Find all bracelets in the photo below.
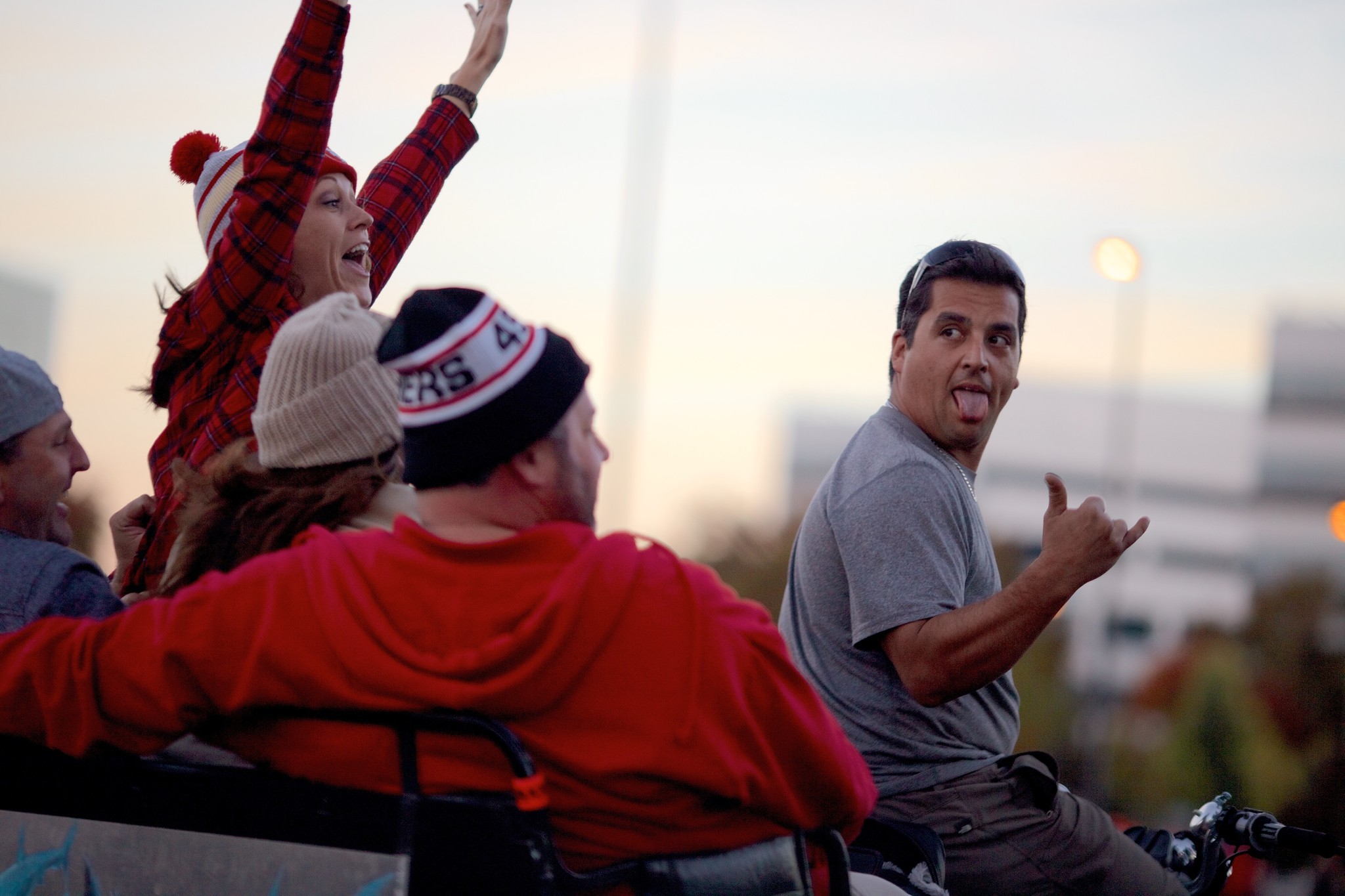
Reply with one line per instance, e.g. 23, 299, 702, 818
431, 83, 478, 118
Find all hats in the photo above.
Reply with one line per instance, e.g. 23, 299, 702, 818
252, 292, 409, 466
0, 349, 62, 442
378, 285, 589, 484
168, 131, 357, 259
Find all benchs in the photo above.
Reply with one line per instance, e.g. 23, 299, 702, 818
0, 701, 853, 896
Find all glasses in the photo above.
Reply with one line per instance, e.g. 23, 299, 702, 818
902, 242, 1025, 329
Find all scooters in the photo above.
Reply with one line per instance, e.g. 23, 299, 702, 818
0, 701, 1345, 896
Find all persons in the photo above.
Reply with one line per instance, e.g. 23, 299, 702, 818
775, 241, 1195, 896
121, 0, 514, 595
1, 348, 125, 632
0, 288, 879, 896
156, 293, 407, 596
